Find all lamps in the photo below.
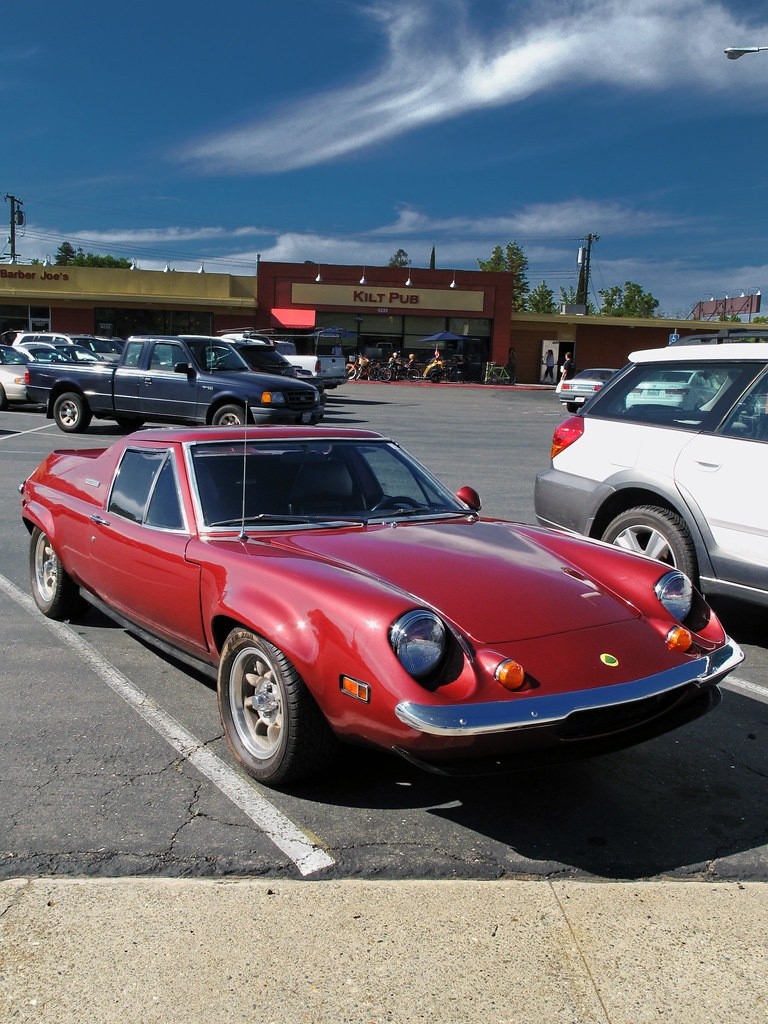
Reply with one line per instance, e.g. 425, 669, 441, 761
405, 268, 413, 287
129, 257, 139, 272
449, 270, 458, 289
197, 261, 206, 275
315, 264, 324, 282
163, 260, 171, 274
358, 266, 368, 285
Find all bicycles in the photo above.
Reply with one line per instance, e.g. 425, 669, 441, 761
480, 363, 516, 386
346, 353, 470, 386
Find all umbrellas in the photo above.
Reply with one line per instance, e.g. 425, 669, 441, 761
311, 324, 357, 355
419, 330, 480, 361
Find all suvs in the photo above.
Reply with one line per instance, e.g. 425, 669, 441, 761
535, 343, 768, 635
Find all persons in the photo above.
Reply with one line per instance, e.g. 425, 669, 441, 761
359, 357, 370, 381
539, 350, 555, 385
560, 352, 574, 403
388, 352, 402, 380
508, 346, 517, 377
3, 326, 17, 347
332, 342, 342, 355
406, 353, 415, 383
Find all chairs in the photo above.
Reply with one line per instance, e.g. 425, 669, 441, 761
284, 460, 368, 516
154, 462, 220, 523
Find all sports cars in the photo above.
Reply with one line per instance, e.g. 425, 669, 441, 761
17, 421, 747, 786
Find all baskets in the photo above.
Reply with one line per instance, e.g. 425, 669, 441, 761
349, 356, 358, 362
359, 357, 369, 366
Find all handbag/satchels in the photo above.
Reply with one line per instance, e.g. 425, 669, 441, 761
555, 377, 564, 394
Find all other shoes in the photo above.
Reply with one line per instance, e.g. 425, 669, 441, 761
562, 402, 567, 406
539, 380, 545, 383
547, 381, 553, 385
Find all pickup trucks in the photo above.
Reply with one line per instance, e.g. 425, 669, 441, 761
25, 335, 325, 434
213, 336, 350, 391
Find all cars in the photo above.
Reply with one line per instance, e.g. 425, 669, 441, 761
0, 328, 160, 372
0, 345, 33, 410
558, 368, 623, 413
625, 368, 722, 417
